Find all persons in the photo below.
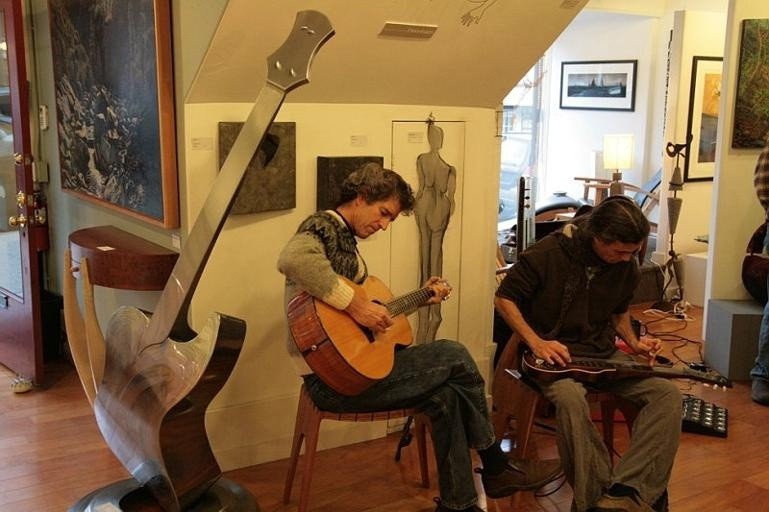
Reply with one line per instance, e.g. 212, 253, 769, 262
412, 124, 457, 345
748, 300, 769, 404
494, 197, 683, 511
276, 160, 563, 512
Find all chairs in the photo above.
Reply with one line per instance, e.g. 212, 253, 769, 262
502, 163, 661, 263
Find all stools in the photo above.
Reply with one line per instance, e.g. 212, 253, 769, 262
282, 385, 434, 512
492, 367, 644, 512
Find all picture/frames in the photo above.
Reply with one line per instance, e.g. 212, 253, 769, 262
559, 60, 637, 112
47, 0, 182, 232
683, 56, 724, 182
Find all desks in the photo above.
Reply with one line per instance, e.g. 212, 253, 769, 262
63, 224, 177, 407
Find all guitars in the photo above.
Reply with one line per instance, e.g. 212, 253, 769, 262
288, 276, 451, 396
94, 10, 334, 511
522, 346, 734, 390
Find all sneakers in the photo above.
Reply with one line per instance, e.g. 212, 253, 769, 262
432, 496, 484, 512
751, 379, 769, 404
474, 457, 561, 499
